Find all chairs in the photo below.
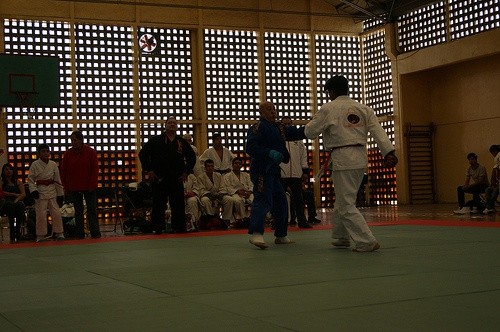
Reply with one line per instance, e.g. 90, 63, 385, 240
95, 186, 125, 234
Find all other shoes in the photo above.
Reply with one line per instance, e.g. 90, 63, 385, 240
308, 218, 322, 224
124, 217, 274, 234
298, 221, 312, 228
291, 221, 297, 226
91, 232, 101, 238
37, 236, 47, 241
53, 233, 65, 240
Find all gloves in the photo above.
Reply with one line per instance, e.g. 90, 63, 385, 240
32, 191, 39, 199
57, 196, 63, 207
385, 153, 398, 168
269, 149, 284, 164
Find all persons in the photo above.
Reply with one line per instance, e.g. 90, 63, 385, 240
59, 131, 101, 239
182, 173, 198, 232
196, 159, 234, 228
0, 164, 27, 243
482, 145, 500, 213
181, 134, 198, 165
304, 75, 398, 252
246, 100, 307, 250
28, 144, 65, 241
138, 117, 196, 234
198, 133, 235, 177
223, 157, 272, 228
287, 169, 321, 225
278, 117, 313, 227
453, 153, 489, 213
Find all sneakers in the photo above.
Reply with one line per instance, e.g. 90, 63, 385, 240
353, 241, 380, 252
481, 208, 496, 214
275, 236, 296, 244
470, 207, 480, 213
453, 207, 465, 214
250, 233, 268, 250
330, 239, 351, 248
